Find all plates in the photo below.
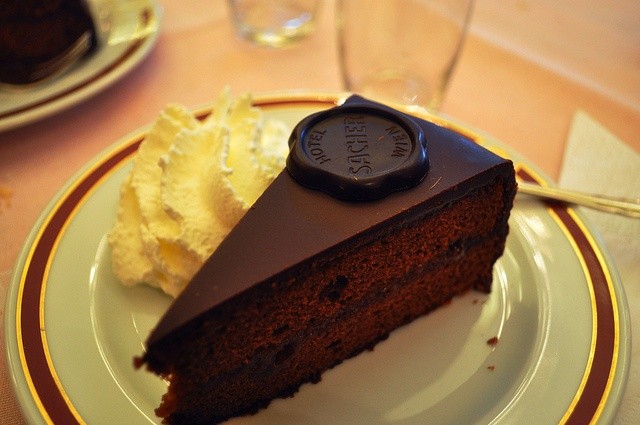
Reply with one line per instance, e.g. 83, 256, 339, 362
0, 0, 163, 135
6, 90, 633, 425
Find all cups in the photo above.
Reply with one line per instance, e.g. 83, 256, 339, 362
334, 1, 473, 118
231, 1, 326, 50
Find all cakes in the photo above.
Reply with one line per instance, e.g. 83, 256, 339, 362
148, 92, 517, 425
